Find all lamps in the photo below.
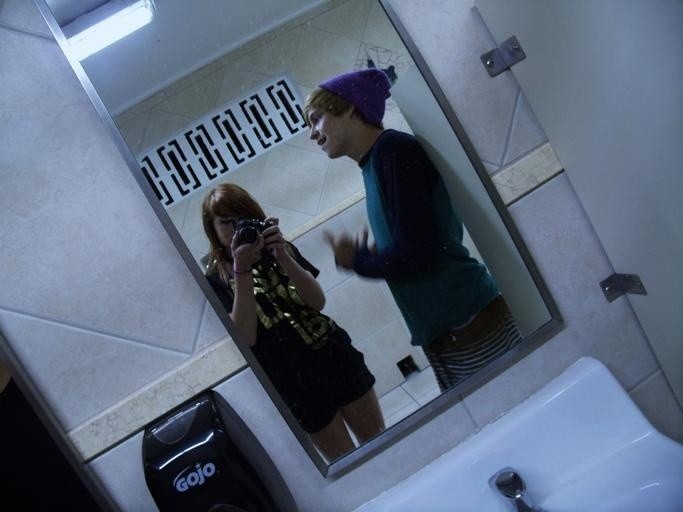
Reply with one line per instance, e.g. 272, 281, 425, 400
60, 0, 155, 63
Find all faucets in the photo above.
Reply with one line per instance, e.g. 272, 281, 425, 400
487, 466, 540, 510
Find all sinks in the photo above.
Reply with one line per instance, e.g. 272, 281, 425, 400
348, 356, 682, 511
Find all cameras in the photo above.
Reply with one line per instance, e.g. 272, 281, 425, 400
235, 221, 270, 247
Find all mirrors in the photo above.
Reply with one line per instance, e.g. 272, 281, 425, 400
33, 0, 565, 482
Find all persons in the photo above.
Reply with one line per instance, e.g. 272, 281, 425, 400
202, 183, 386, 463
301, 65, 524, 394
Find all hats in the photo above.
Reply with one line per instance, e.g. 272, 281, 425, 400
319, 68, 391, 123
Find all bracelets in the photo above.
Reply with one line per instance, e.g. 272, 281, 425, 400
233, 267, 253, 274
